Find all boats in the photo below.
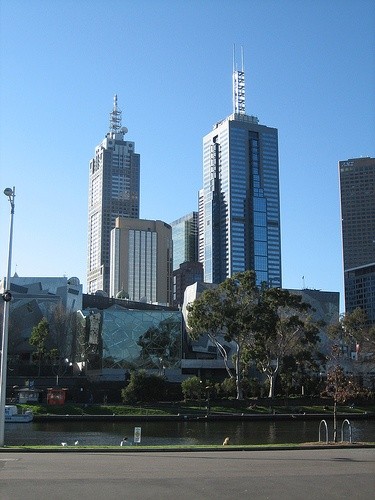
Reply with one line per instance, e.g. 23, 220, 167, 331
5, 405, 35, 422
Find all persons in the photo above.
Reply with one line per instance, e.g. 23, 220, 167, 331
120, 437, 129, 446
222, 435, 232, 445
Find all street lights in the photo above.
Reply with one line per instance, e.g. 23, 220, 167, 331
1, 185, 16, 448
